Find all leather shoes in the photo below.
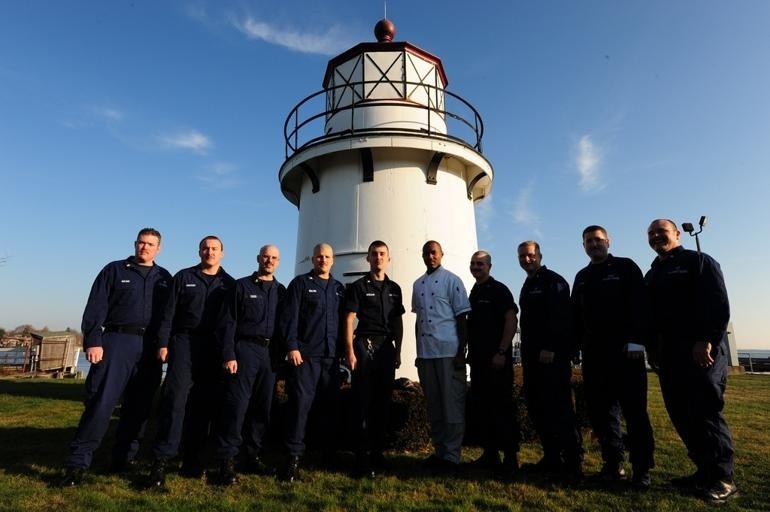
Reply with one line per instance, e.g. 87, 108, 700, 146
60, 456, 304, 490
590, 463, 740, 505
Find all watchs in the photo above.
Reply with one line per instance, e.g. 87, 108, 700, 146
498, 348, 506, 355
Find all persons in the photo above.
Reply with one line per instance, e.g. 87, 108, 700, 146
517, 242, 582, 483
62, 227, 174, 489
218, 243, 287, 485
643, 218, 738, 506
570, 226, 655, 488
344, 242, 405, 479
411, 241, 474, 475
463, 250, 518, 480
137, 235, 236, 491
280, 242, 347, 483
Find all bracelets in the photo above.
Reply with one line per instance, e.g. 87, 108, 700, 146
500, 343, 508, 352
